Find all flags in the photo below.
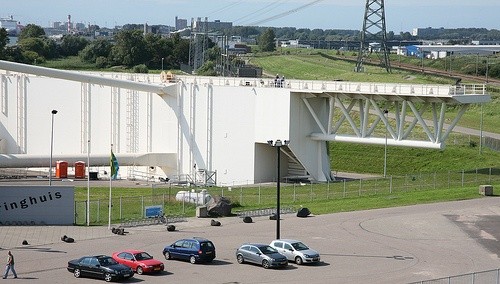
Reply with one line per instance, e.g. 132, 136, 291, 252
110, 147, 120, 180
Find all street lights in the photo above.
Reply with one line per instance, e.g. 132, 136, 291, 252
266, 140, 290, 238
383, 109, 389, 178
48, 110, 58, 185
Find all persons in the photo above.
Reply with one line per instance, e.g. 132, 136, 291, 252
3, 251, 17, 279
274, 74, 285, 87
456, 78, 462, 88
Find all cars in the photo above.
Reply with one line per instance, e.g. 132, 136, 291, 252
162, 237, 217, 265
66, 255, 135, 282
269, 239, 321, 265
236, 243, 288, 270
111, 249, 165, 274
